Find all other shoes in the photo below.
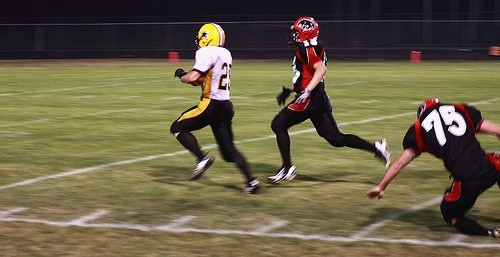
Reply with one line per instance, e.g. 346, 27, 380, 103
488, 227, 500, 238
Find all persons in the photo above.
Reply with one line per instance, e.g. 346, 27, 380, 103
366, 98, 500, 238
267, 16, 392, 184
170, 24, 262, 194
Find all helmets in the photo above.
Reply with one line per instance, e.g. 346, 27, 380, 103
197, 23, 225, 48
417, 98, 439, 119
289, 16, 319, 43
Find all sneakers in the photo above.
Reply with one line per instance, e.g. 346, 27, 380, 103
374, 138, 391, 167
241, 176, 263, 194
267, 164, 297, 186
188, 151, 214, 181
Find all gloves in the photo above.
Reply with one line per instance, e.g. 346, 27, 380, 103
276, 86, 291, 107
295, 88, 311, 105
174, 68, 187, 78
